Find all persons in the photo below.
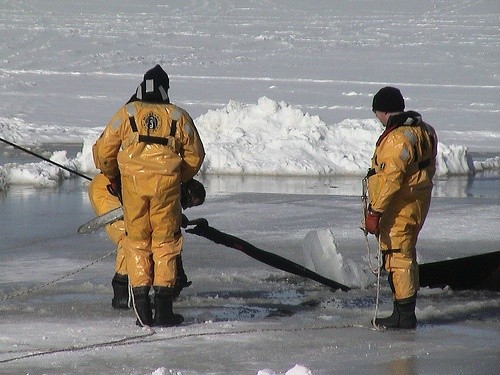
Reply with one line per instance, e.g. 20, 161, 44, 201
92, 64, 205, 327
88, 172, 206, 309
365, 86, 438, 330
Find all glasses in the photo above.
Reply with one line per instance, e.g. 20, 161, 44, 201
372, 109, 377, 114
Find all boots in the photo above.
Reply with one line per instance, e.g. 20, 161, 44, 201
132, 286, 154, 327
371, 292, 417, 329
154, 286, 184, 327
112, 273, 129, 309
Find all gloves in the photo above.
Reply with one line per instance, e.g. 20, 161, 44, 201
365, 209, 382, 237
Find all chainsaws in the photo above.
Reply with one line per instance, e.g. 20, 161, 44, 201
78, 178, 206, 236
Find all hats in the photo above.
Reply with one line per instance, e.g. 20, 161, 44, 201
373, 87, 405, 113
144, 64, 170, 89
